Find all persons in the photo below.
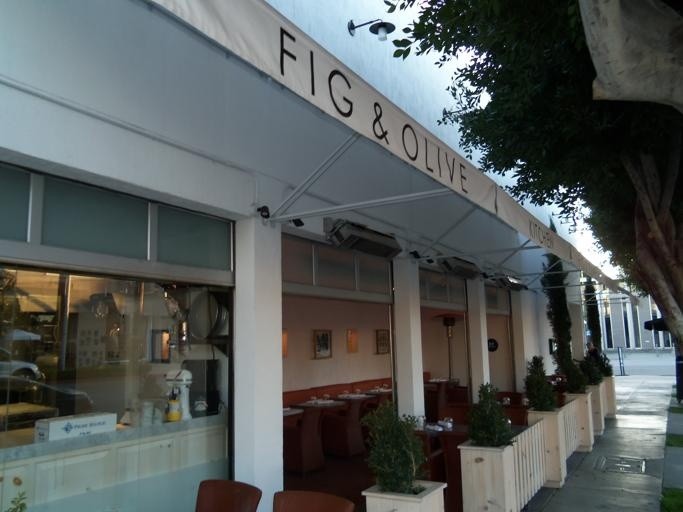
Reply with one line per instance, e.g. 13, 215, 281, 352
585, 343, 599, 359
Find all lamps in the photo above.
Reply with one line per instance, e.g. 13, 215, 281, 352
323, 217, 528, 292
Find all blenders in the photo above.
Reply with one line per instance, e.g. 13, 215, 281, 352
164, 369, 192, 420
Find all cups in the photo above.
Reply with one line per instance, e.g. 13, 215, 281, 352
555, 377, 561, 382
443, 416, 453, 431
502, 397, 512, 405
411, 415, 426, 431
521, 397, 529, 407
165, 400, 181, 422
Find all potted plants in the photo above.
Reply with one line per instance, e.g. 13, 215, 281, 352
359, 400, 447, 512
457, 349, 616, 512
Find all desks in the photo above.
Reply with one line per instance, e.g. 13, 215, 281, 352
1, 402, 59, 451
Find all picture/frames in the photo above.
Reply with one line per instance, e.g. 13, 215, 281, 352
375, 329, 390, 354
313, 329, 332, 359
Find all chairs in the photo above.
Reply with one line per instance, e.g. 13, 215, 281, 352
437, 431, 470, 512
196, 480, 355, 512
